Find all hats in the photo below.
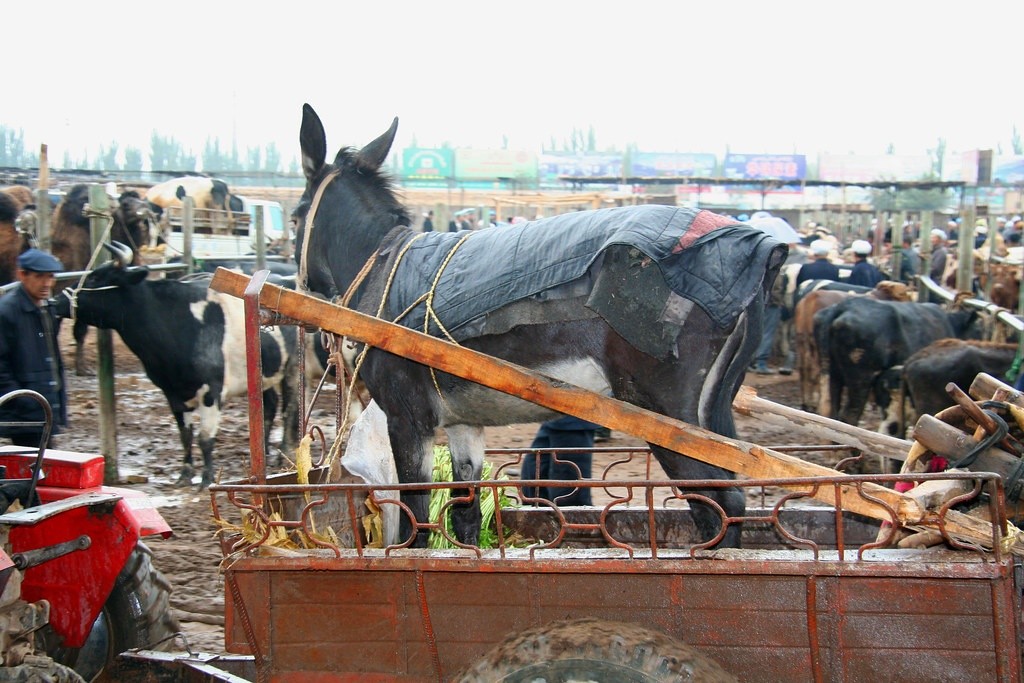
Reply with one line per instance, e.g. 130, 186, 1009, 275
19, 249, 66, 272
931, 229, 948, 242
810, 239, 832, 255
996, 217, 1007, 223
464, 208, 475, 214
975, 226, 987, 234
488, 210, 496, 216
453, 210, 463, 217
975, 218, 988, 227
851, 239, 873, 257
1012, 216, 1021, 223
428, 210, 434, 216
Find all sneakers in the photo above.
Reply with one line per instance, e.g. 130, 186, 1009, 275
755, 367, 774, 374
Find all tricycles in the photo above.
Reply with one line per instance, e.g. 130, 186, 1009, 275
0, 269, 1024, 683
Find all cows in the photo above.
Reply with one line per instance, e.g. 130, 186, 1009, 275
770, 252, 1024, 466
46, 239, 360, 490
143, 175, 243, 250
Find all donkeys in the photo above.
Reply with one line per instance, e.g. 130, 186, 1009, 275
0, 182, 162, 295
289, 101, 779, 549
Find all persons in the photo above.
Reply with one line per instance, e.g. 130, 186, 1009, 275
422, 209, 435, 232
518, 411, 603, 506
795, 238, 840, 290
446, 209, 516, 232
868, 214, 1024, 291
848, 239, 888, 287
0, 249, 68, 449
